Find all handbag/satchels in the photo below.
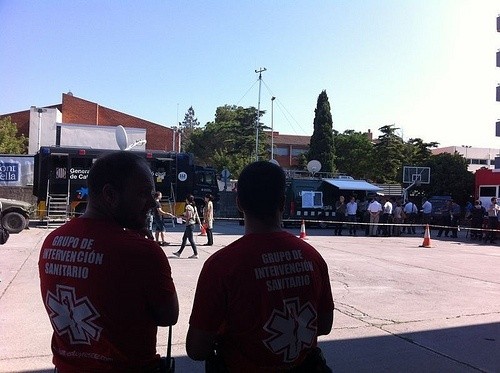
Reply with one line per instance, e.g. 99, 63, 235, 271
401, 211, 407, 218
411, 212, 418, 218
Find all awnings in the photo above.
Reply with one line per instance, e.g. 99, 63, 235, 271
322, 178, 384, 191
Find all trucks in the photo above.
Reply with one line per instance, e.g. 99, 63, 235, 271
285, 177, 385, 227
33, 144, 219, 224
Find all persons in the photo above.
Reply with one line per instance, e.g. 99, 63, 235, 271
334, 194, 500, 244
198, 194, 215, 246
186, 161, 335, 373
153, 191, 173, 245
40, 151, 178, 373
173, 194, 204, 258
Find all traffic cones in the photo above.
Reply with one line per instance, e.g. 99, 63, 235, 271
419, 224, 432, 248
197, 223, 207, 236
299, 220, 309, 240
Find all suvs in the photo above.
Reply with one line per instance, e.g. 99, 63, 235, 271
0, 194, 36, 234
424, 195, 454, 224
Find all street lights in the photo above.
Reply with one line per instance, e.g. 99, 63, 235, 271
270, 96, 277, 159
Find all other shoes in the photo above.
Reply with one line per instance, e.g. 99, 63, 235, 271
338, 233, 342, 236
413, 232, 415, 234
162, 242, 170, 246
490, 240, 496, 244
334, 233, 337, 236
445, 235, 449, 237
173, 252, 180, 256
484, 240, 487, 243
408, 232, 411, 234
450, 235, 457, 238
437, 235, 441, 237
188, 255, 199, 259
204, 244, 212, 246
